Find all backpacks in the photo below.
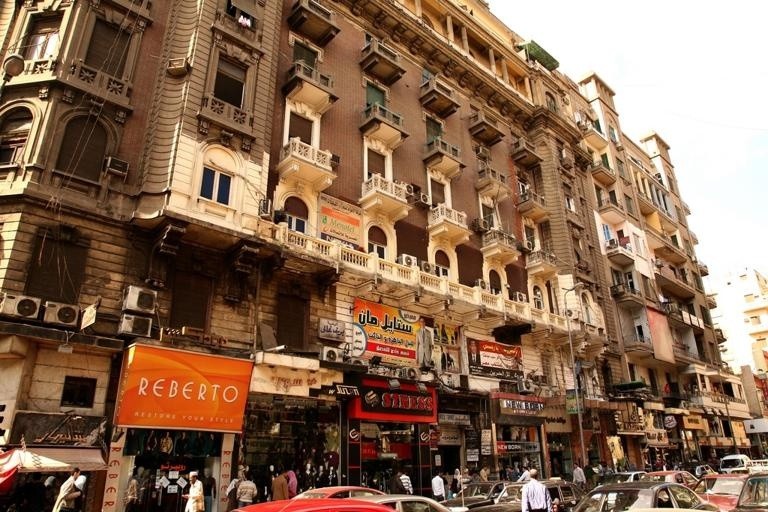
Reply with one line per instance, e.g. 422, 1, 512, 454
389, 474, 407, 494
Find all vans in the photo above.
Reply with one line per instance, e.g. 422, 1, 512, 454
720, 454, 751, 474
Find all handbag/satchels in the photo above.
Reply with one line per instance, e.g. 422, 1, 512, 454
229, 487, 236, 500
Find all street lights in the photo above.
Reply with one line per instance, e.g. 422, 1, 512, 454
715, 349, 740, 454
564, 282, 586, 469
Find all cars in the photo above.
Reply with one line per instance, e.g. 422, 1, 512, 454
229, 485, 451, 512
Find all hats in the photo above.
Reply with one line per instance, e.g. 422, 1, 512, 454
189, 470, 199, 477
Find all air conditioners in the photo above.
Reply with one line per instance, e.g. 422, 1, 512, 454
520, 238, 535, 253
473, 277, 491, 293
476, 145, 490, 158
415, 190, 434, 208
106, 155, 130, 178
420, 258, 436, 275
0, 284, 158, 338
397, 253, 420, 269
607, 239, 677, 313
322, 345, 534, 393
167, 57, 189, 75
474, 216, 491, 233
397, 181, 414, 198
511, 290, 528, 304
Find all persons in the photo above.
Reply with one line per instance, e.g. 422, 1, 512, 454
224, 463, 338, 507
431, 466, 539, 501
572, 462, 586, 489
201, 468, 216, 512
655, 457, 686, 471
182, 471, 206, 512
390, 469, 414, 496
37, 468, 87, 511
585, 460, 631, 482
522, 470, 553, 512
